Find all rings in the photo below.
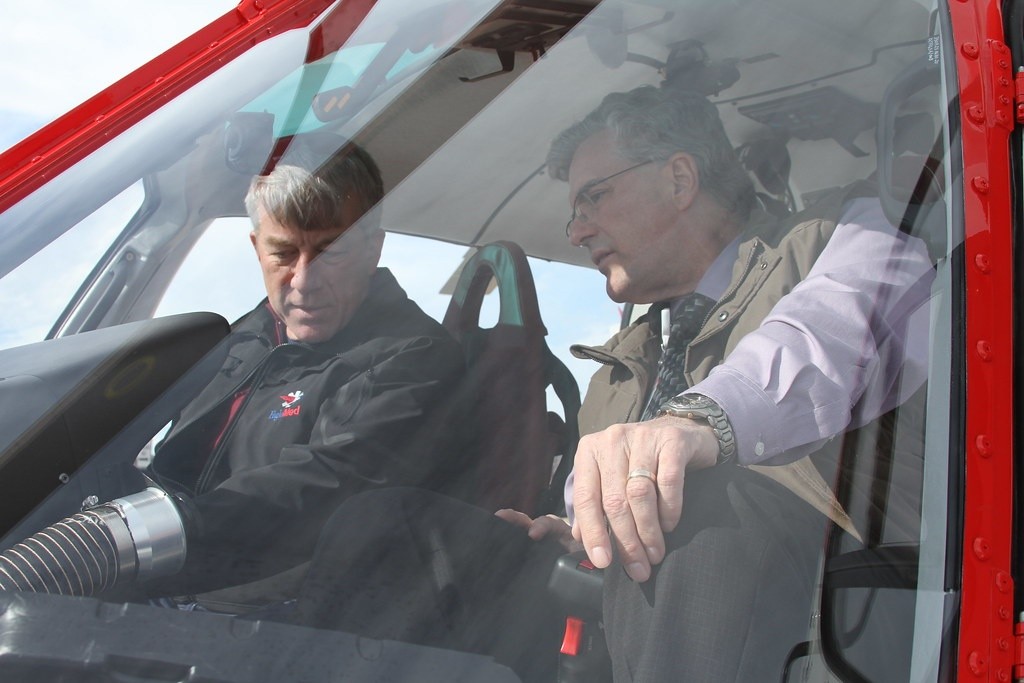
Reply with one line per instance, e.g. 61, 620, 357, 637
626, 470, 657, 483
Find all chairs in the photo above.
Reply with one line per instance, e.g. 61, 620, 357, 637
421, 240, 581, 520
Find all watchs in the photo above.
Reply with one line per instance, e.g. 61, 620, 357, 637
656, 393, 737, 464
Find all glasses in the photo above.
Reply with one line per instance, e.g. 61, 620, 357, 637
566, 160, 657, 237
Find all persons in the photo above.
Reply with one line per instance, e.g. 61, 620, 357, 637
289, 85, 937, 683
60, 130, 484, 626
